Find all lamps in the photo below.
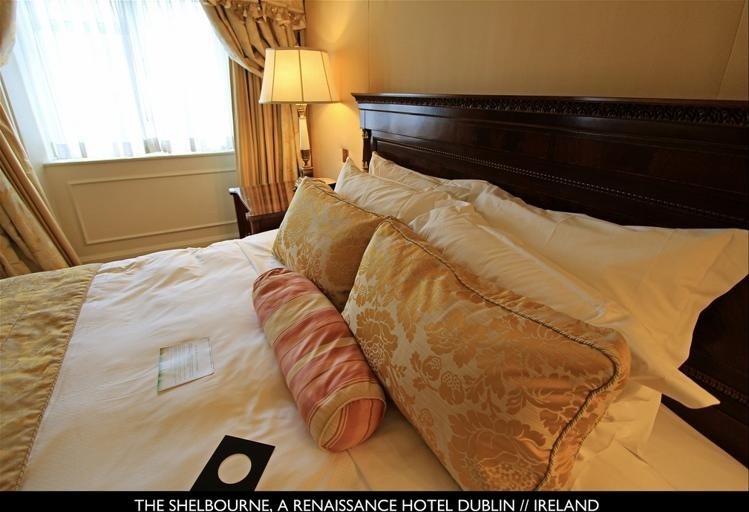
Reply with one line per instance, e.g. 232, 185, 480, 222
256, 47, 339, 186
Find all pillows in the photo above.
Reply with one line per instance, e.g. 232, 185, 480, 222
368, 150, 487, 203
465, 177, 748, 466
250, 264, 386, 453
271, 176, 384, 314
342, 222, 632, 491
330, 157, 454, 225
402, 194, 723, 491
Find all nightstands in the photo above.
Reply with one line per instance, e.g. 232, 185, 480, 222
226, 179, 296, 240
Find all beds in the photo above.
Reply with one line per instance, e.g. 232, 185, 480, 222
1, 94, 747, 490
